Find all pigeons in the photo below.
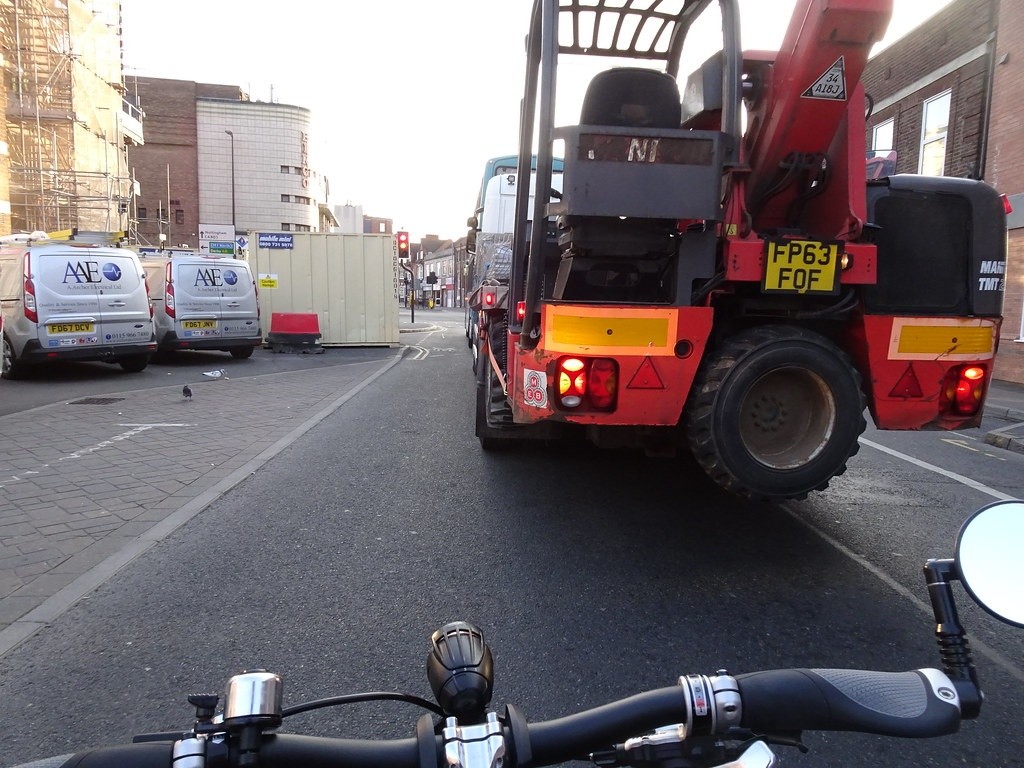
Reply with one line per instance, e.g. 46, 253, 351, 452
182, 384, 192, 401
201, 368, 226, 380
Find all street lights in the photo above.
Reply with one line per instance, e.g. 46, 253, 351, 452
225, 129, 235, 225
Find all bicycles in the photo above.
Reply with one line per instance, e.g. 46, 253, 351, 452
11, 504, 1024, 768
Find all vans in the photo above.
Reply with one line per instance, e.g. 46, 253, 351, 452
135, 251, 263, 358
0, 242, 158, 380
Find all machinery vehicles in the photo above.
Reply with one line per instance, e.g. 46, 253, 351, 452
465, 0, 1005, 503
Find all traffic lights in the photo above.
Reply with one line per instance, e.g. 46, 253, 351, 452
436, 277, 438, 283
427, 276, 430, 284
404, 277, 406, 284
398, 232, 408, 257
